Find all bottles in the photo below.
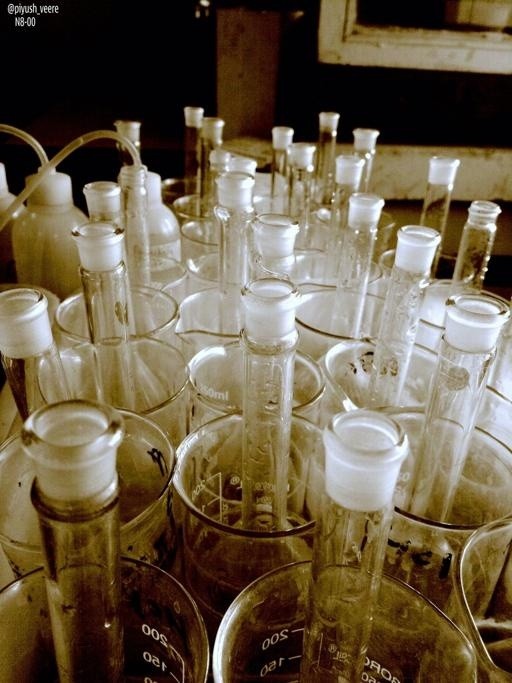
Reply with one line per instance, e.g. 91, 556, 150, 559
0, 99, 512, 681
